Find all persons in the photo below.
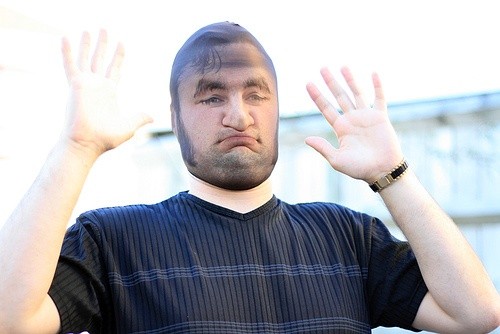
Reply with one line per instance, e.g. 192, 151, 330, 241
1, 19, 499, 333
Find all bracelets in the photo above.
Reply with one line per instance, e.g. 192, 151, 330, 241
370, 155, 409, 195
